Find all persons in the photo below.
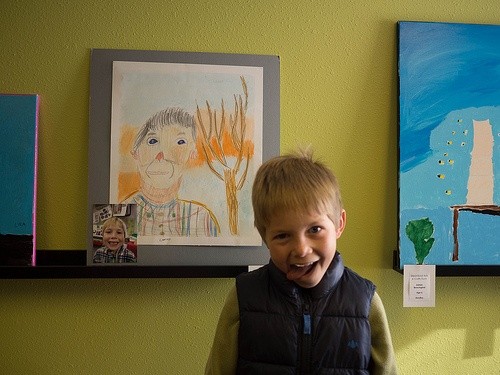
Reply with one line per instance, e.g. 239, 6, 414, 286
92, 216, 136, 263
204, 138, 394, 375
